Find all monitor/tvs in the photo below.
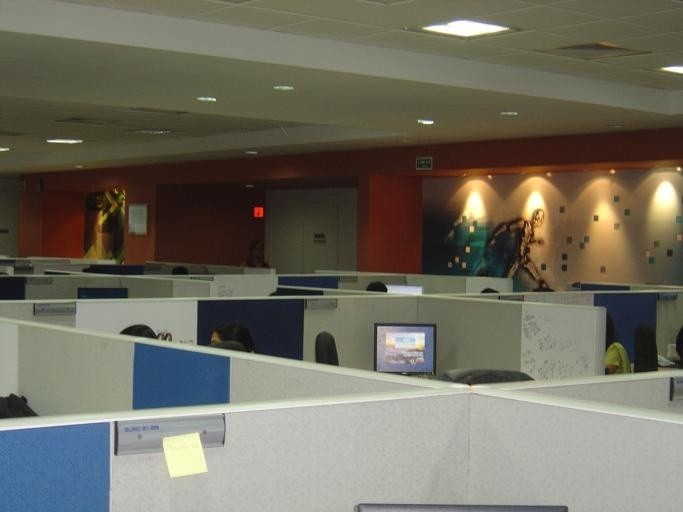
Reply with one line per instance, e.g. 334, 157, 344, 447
77, 287, 127, 299
0, 277, 27, 300
373, 321, 436, 375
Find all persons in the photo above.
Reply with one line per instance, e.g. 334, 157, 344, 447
210, 322, 256, 353
172, 266, 190, 275
481, 287, 499, 293
241, 238, 271, 269
366, 281, 387, 292
120, 324, 157, 339
487, 209, 550, 289
604, 312, 632, 375
